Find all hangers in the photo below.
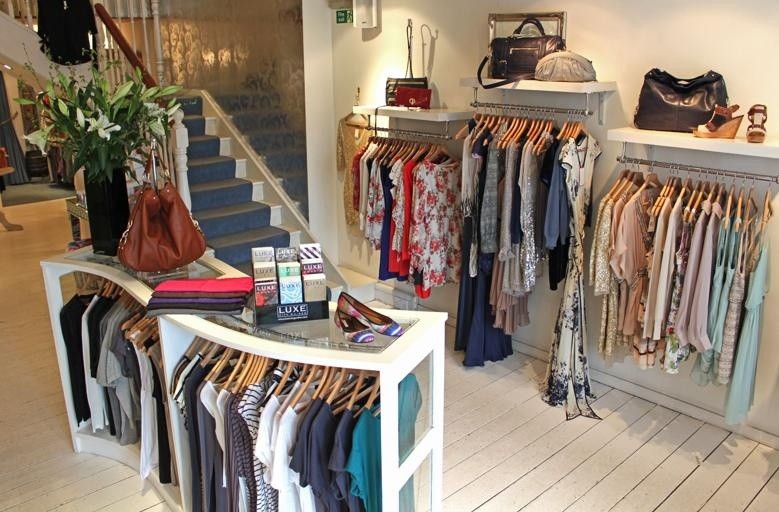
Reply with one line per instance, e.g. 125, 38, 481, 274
76, 274, 160, 359
343, 87, 368, 130
607, 156, 775, 236
365, 130, 451, 165
454, 102, 589, 157
166, 335, 379, 416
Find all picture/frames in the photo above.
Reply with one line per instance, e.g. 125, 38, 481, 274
488, 11, 567, 77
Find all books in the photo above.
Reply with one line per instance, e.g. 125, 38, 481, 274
251, 243, 327, 307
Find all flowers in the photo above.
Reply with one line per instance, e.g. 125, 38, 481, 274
6, 30, 184, 183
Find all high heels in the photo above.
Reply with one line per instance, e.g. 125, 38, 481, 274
692, 102, 744, 139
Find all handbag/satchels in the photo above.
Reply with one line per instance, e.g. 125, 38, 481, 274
116, 147, 209, 275
632, 62, 731, 135
382, 74, 433, 112
474, 16, 598, 89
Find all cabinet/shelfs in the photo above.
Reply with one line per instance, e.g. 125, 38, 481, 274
38, 240, 449, 511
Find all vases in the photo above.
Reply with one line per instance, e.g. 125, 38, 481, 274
83, 169, 136, 254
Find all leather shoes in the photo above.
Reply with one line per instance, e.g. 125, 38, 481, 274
332, 291, 404, 346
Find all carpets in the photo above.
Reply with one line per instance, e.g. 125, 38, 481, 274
2, 181, 77, 207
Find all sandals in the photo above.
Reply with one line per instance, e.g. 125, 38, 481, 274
744, 102, 769, 144
